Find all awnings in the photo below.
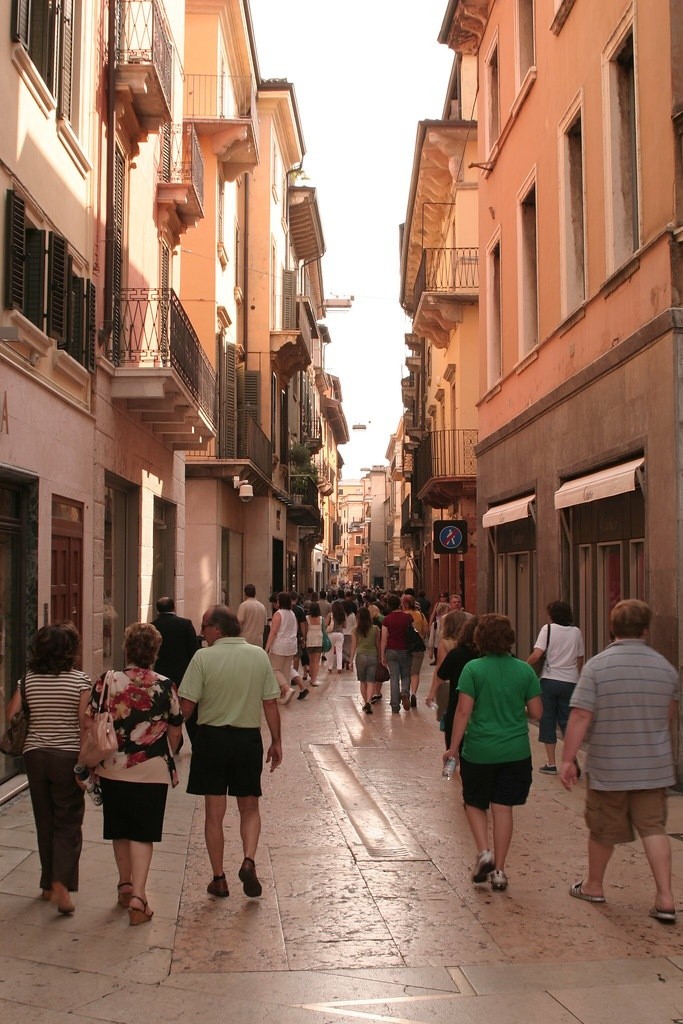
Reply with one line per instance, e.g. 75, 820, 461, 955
482, 494, 536, 550
554, 456, 645, 543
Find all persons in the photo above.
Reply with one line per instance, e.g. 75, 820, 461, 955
151, 597, 199, 753
526, 600, 585, 779
103, 590, 118, 655
443, 614, 544, 892
75, 623, 183, 926
265, 592, 298, 705
7, 620, 92, 912
178, 604, 282, 897
437, 615, 481, 757
560, 600, 680, 919
268, 580, 473, 714
426, 610, 467, 722
237, 584, 266, 649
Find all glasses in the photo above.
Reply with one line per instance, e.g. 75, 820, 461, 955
201, 622, 220, 631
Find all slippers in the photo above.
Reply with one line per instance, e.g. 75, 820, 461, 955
569, 879, 606, 901
650, 906, 677, 921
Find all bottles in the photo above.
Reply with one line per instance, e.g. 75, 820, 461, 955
442, 755, 455, 781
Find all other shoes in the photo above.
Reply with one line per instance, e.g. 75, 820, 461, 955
175, 739, 183, 754
361, 690, 417, 716
42, 889, 52, 900
52, 881, 75, 913
278, 661, 350, 705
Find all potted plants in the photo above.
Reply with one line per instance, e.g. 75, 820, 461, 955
291, 477, 308, 505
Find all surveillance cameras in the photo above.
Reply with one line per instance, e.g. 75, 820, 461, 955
239, 484, 254, 502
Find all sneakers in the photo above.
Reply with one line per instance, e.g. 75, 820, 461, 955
471, 847, 494, 882
539, 764, 557, 775
574, 756, 581, 778
490, 869, 507, 892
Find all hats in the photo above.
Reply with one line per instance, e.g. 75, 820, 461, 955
415, 602, 421, 609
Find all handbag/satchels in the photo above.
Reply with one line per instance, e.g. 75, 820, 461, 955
0, 674, 30, 756
375, 663, 389, 682
322, 617, 332, 652
531, 623, 551, 677
77, 670, 118, 768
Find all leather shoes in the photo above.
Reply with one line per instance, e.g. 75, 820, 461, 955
207, 879, 229, 897
239, 860, 262, 897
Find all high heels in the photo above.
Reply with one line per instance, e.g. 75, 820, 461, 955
117, 882, 133, 908
128, 896, 153, 925
